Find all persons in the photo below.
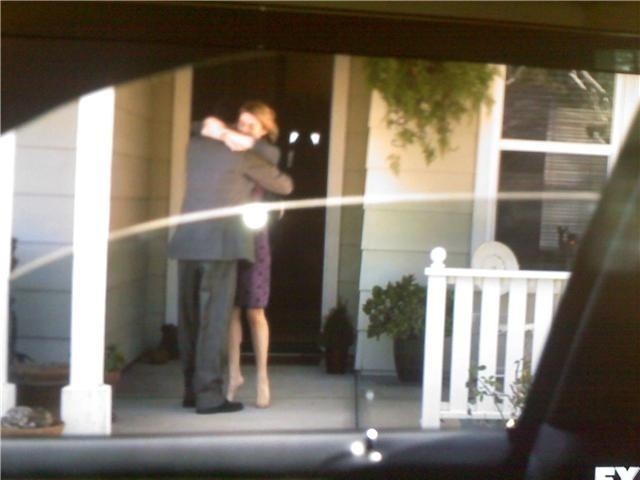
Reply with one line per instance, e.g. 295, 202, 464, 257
162, 100, 295, 415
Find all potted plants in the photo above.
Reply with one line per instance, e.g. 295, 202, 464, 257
104, 343, 126, 393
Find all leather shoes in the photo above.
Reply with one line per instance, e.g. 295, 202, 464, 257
184, 399, 243, 414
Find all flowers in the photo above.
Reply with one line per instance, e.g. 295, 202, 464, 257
317, 301, 354, 346
362, 275, 454, 340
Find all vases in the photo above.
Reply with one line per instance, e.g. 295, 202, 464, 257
327, 347, 348, 372
395, 339, 424, 383
